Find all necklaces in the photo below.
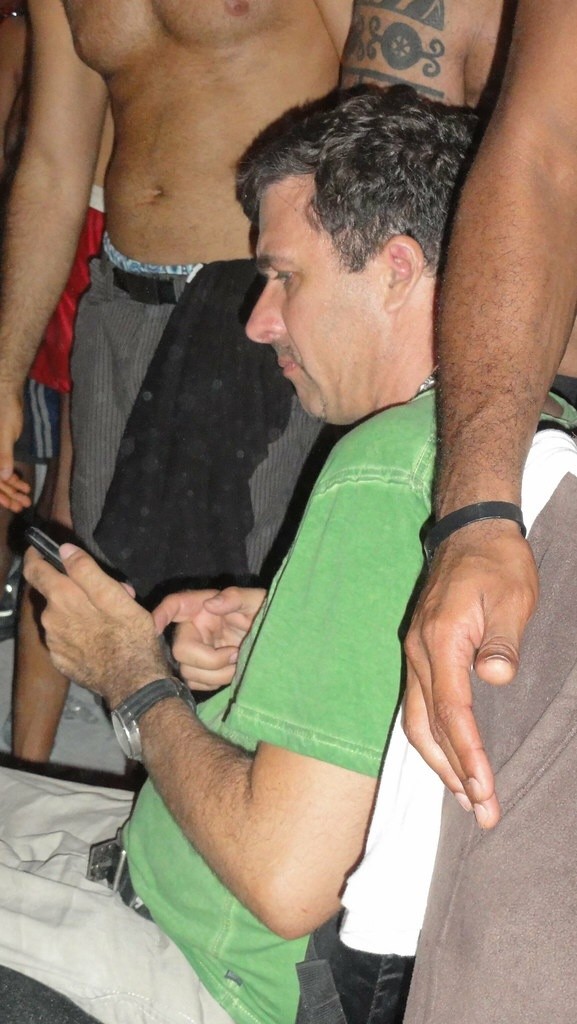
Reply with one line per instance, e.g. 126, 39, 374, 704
418, 377, 435, 392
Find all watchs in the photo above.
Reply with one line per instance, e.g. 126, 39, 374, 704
111, 677, 196, 761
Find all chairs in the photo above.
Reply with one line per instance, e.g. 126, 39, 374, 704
0, 420, 577, 1024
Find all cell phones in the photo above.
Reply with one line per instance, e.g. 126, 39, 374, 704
25, 527, 69, 575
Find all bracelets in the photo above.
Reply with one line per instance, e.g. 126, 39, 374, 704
423, 501, 526, 559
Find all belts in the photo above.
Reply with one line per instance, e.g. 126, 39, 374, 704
87, 828, 155, 921
99, 247, 188, 305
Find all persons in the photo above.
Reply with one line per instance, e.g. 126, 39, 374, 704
21, 84, 576, 1024
0, 0, 355, 611
340, 0, 513, 118
401, 0, 576, 826
0, 15, 115, 764
